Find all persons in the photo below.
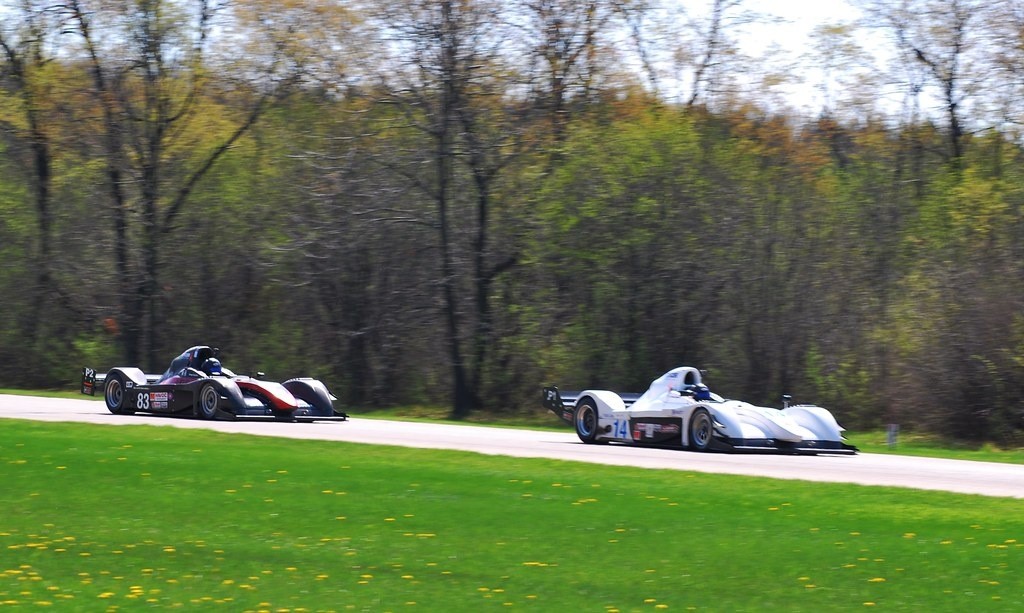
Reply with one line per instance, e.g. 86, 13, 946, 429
201, 357, 221, 376
693, 383, 709, 401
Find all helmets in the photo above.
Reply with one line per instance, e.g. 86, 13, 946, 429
692, 383, 710, 402
200, 358, 222, 376
